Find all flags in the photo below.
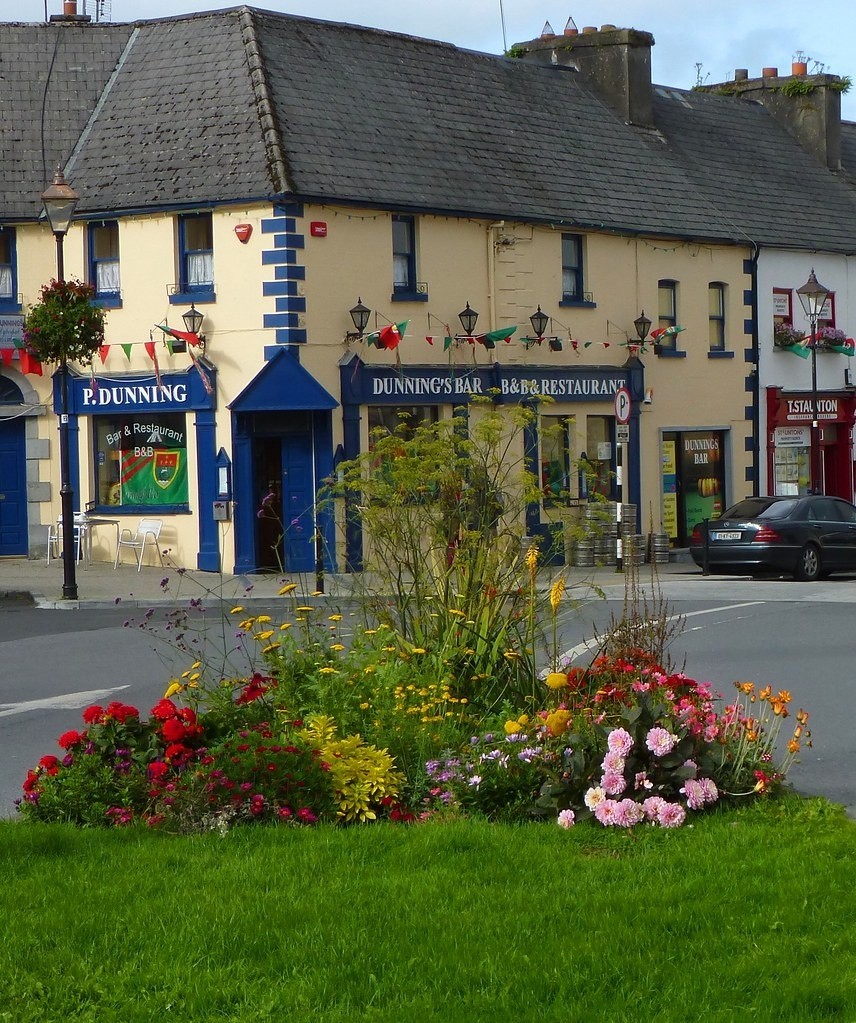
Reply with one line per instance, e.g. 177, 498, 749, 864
119, 448, 189, 506
11, 337, 42, 377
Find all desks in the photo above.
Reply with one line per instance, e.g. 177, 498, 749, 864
54, 517, 121, 571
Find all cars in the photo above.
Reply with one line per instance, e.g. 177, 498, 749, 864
688, 495, 856, 582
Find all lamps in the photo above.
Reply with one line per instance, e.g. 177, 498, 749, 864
526, 305, 550, 351
454, 300, 479, 348
182, 302, 206, 350
630, 309, 652, 346
347, 297, 371, 343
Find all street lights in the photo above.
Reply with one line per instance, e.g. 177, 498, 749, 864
38, 162, 81, 602
796, 267, 831, 493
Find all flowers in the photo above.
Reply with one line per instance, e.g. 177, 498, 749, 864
774, 322, 805, 347
820, 326, 847, 343
20, 277, 109, 366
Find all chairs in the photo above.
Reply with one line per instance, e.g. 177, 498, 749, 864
47, 512, 90, 565
114, 518, 165, 572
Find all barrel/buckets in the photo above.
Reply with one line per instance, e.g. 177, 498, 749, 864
651, 533, 670, 562
516, 537, 535, 569
571, 501, 645, 567
533, 522, 565, 566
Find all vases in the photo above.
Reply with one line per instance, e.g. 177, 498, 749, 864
829, 340, 841, 346
